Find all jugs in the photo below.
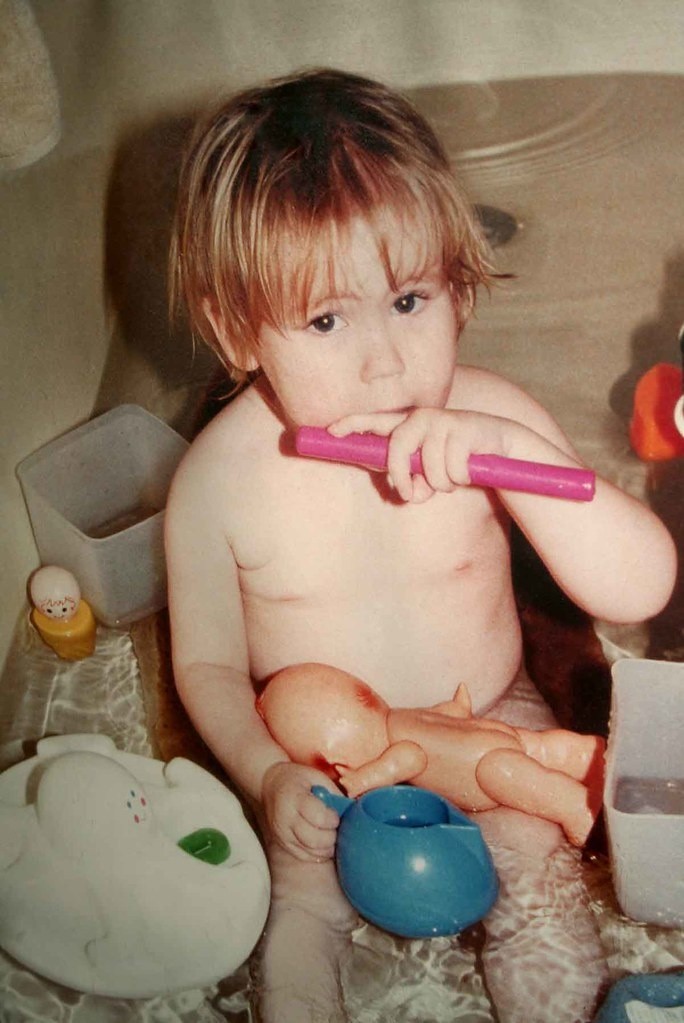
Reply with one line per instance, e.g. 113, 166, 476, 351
310, 780, 501, 938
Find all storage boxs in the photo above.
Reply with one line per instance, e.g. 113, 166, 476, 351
16, 403, 191, 627
595, 618, 684, 928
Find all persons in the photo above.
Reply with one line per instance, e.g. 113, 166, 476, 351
255, 662, 605, 849
162, 68, 677, 861
